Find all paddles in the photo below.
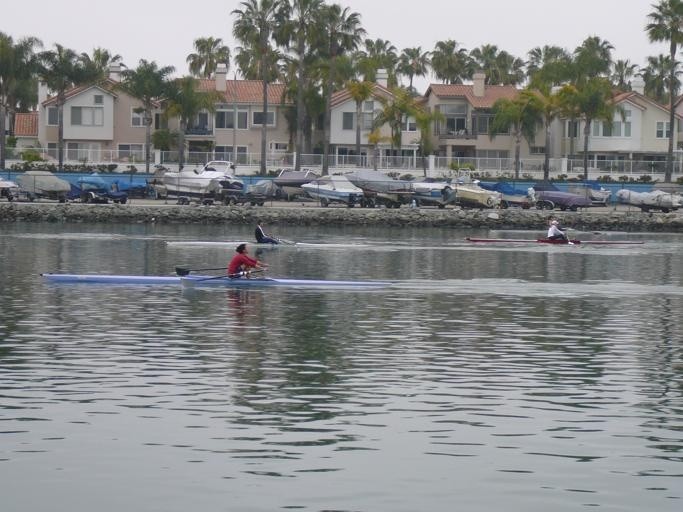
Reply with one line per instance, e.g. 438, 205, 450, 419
182, 270, 269, 288
175, 264, 270, 276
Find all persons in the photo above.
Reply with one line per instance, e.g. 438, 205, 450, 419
255, 220, 284, 244
547, 220, 574, 245
227, 243, 268, 279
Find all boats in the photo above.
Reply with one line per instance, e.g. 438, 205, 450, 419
221, 166, 613, 211
40, 269, 393, 287
467, 235, 645, 244
162, 237, 374, 249
615, 188, 683, 212
0, 170, 174, 204
163, 161, 245, 205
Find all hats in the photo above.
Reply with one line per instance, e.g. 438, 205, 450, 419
551, 220, 559, 225
